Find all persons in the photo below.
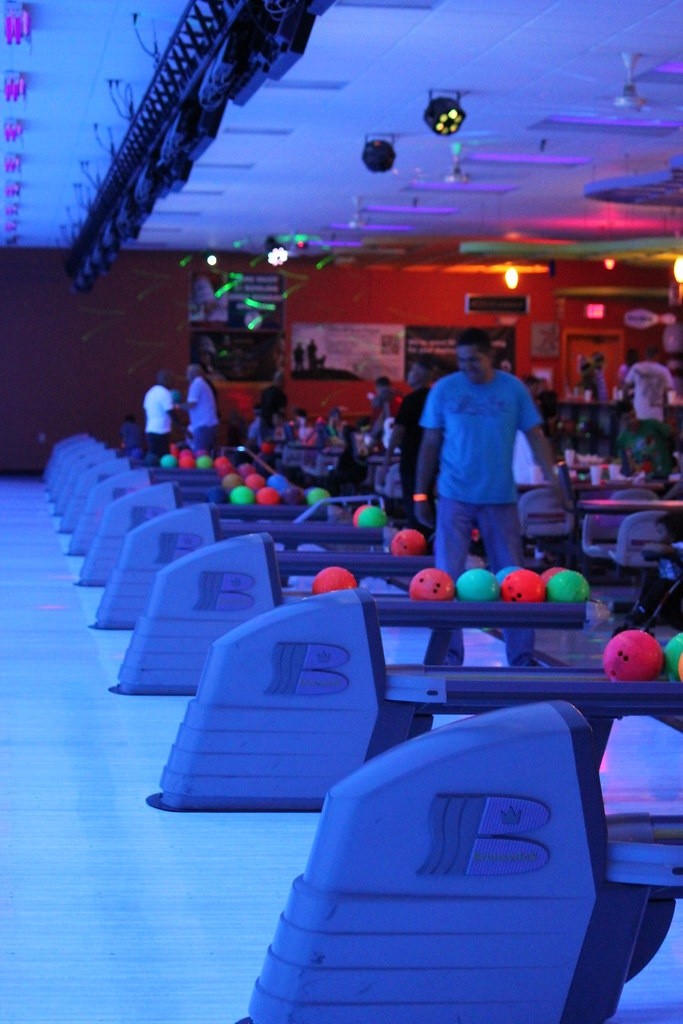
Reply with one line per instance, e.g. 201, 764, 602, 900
577, 347, 682, 409
624, 511, 682, 629
619, 343, 676, 423
611, 399, 675, 485
410, 326, 579, 672
380, 359, 443, 531
117, 336, 404, 481
660, 442, 682, 503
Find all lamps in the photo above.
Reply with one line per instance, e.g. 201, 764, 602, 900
60, 0, 336, 299
362, 133, 396, 172
423, 89, 466, 136
614, 52, 651, 112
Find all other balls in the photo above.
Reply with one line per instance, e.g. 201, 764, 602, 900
160, 444, 681, 683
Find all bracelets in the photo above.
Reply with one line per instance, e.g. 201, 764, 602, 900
410, 492, 428, 502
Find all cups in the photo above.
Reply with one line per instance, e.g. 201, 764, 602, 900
564, 450, 575, 468
590, 465, 603, 486
608, 464, 622, 481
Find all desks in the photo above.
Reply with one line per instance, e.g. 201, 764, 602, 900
556, 398, 632, 455
578, 497, 683, 626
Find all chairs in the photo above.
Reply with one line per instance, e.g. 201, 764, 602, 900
283, 437, 671, 613
